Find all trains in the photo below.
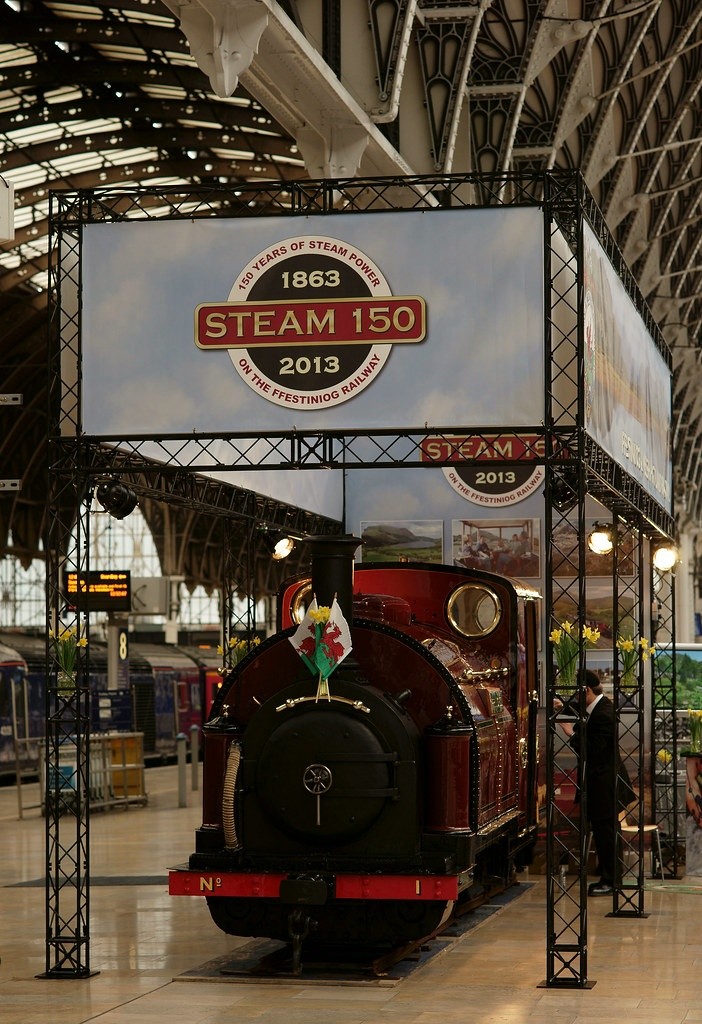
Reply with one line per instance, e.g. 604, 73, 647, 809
167, 534, 577, 971
0, 631, 238, 780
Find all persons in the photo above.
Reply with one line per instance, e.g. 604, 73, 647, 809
552, 668, 637, 897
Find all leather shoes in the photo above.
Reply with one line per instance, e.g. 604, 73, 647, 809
588, 882, 620, 896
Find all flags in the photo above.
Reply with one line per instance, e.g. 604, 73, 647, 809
321, 599, 353, 682
288, 598, 325, 675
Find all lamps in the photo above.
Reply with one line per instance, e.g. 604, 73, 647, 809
652, 537, 678, 571
96, 481, 138, 520
261, 525, 294, 560
542, 473, 587, 512
587, 520, 623, 556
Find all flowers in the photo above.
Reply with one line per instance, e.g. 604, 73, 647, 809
658, 745, 689, 762
216, 636, 261, 669
616, 635, 658, 671
308, 606, 332, 635
47, 624, 89, 672
687, 709, 702, 736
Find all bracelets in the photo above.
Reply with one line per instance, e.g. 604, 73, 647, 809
569, 732, 576, 738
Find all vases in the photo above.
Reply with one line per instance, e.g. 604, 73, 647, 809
549, 620, 600, 670
689, 736, 702, 752
618, 672, 637, 692
56, 672, 78, 696
556, 669, 578, 696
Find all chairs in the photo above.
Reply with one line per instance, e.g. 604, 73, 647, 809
583, 809, 665, 882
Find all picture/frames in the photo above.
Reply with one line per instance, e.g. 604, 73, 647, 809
552, 586, 635, 651
553, 660, 637, 712
360, 519, 443, 565
551, 516, 636, 578
452, 518, 541, 579
517, 586, 541, 652
